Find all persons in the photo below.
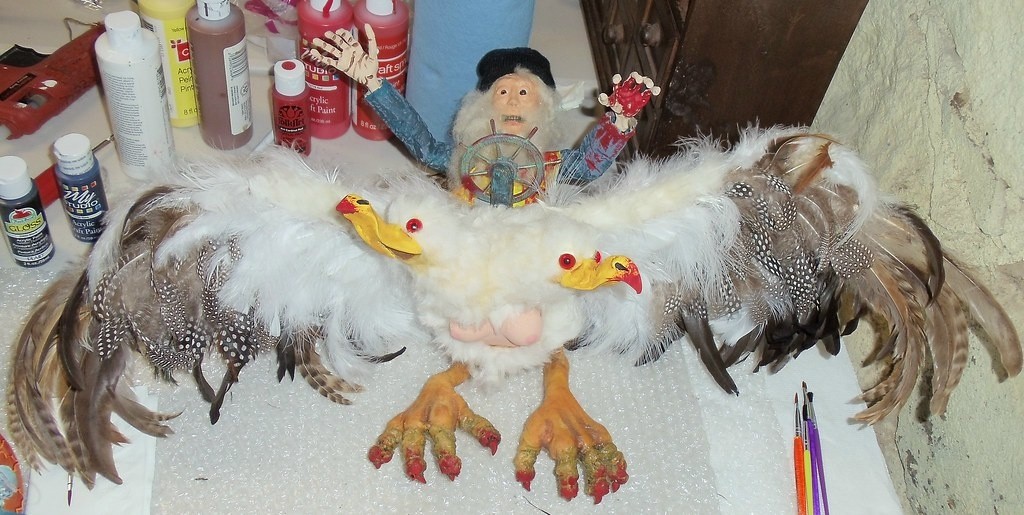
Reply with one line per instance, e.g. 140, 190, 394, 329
309, 24, 660, 209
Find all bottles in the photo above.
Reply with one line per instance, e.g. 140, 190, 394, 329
351, 0, 408, 141
53, 134, 110, 243
0, 156, 55, 266
184, 0, 253, 149
272, 59, 311, 157
94, 10, 176, 180
297, 0, 351, 140
137, 0, 199, 128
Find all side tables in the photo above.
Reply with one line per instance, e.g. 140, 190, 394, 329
577, 0, 870, 173
